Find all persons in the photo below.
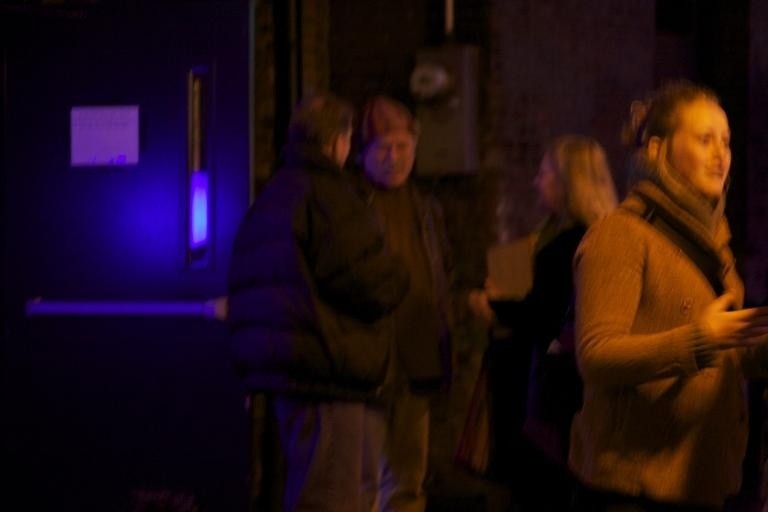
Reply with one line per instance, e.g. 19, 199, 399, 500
570, 77, 768, 511
353, 91, 456, 512
228, 90, 411, 511
465, 131, 621, 511
